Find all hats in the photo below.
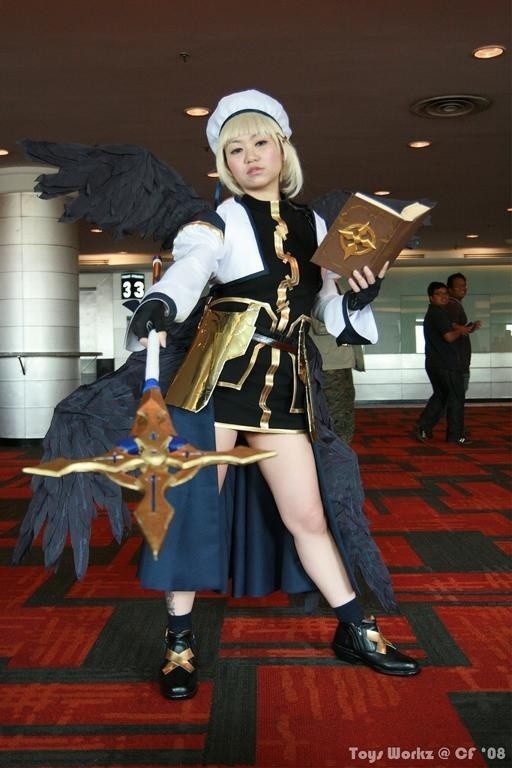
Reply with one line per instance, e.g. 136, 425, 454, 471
205, 90, 292, 154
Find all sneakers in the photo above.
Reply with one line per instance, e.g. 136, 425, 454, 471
413, 426, 428, 440
447, 437, 472, 446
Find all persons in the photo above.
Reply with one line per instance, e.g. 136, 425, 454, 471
439, 273, 481, 416
307, 320, 365, 446
126, 87, 423, 700
414, 282, 471, 446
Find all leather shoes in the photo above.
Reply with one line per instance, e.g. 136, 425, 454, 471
330, 613, 421, 676
160, 628, 200, 700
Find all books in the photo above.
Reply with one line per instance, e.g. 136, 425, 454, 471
310, 192, 438, 285
